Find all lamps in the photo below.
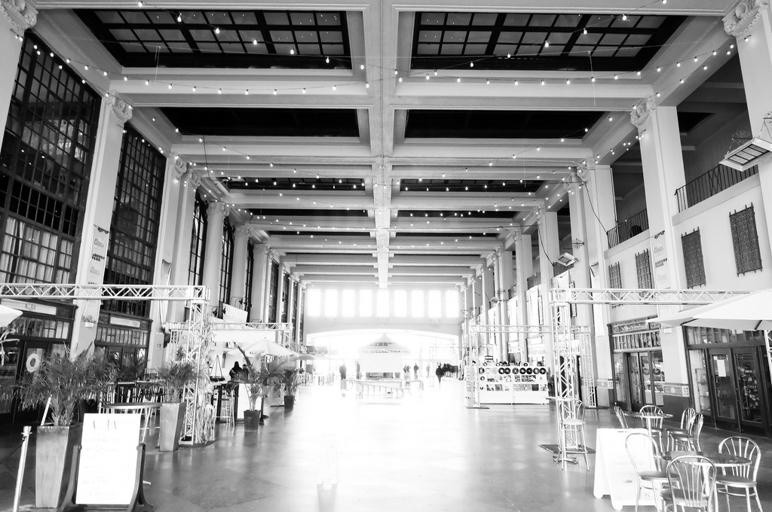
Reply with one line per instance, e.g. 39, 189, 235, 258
718, 112, 772, 173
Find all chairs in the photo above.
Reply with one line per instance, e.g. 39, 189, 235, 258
555, 398, 590, 471
613, 404, 763, 512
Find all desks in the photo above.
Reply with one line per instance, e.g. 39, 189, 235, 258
595, 428, 663, 512
103, 402, 162, 444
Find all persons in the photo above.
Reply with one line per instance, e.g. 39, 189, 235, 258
355, 359, 361, 382
413, 362, 420, 380
338, 361, 348, 381
435, 365, 444, 382
229, 360, 245, 382
241, 363, 250, 379
472, 358, 543, 367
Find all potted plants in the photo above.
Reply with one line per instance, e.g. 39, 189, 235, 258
0, 341, 123, 508
154, 362, 208, 452
224, 341, 314, 430
